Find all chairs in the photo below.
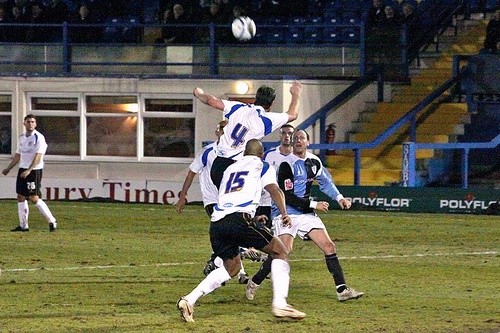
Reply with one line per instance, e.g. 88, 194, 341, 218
249, 0, 490, 68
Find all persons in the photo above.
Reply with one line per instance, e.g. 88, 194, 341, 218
0, 0, 500, 61
176, 138, 307, 323
245, 130, 365, 302
175, 81, 303, 287
2, 114, 57, 233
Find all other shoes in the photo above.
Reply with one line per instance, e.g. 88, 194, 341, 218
238, 273, 250, 284
49, 218, 57, 232
11, 226, 29, 232
261, 256, 270, 278
203, 260, 225, 286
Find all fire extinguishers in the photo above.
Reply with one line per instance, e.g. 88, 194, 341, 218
324, 122, 336, 155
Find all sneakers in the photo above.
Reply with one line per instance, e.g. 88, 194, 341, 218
336, 286, 364, 302
176, 297, 195, 323
271, 303, 306, 319
246, 277, 260, 301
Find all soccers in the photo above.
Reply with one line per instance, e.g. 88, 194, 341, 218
232, 16, 257, 41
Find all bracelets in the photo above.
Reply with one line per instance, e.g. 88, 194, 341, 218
335, 194, 344, 203
310, 201, 317, 210
